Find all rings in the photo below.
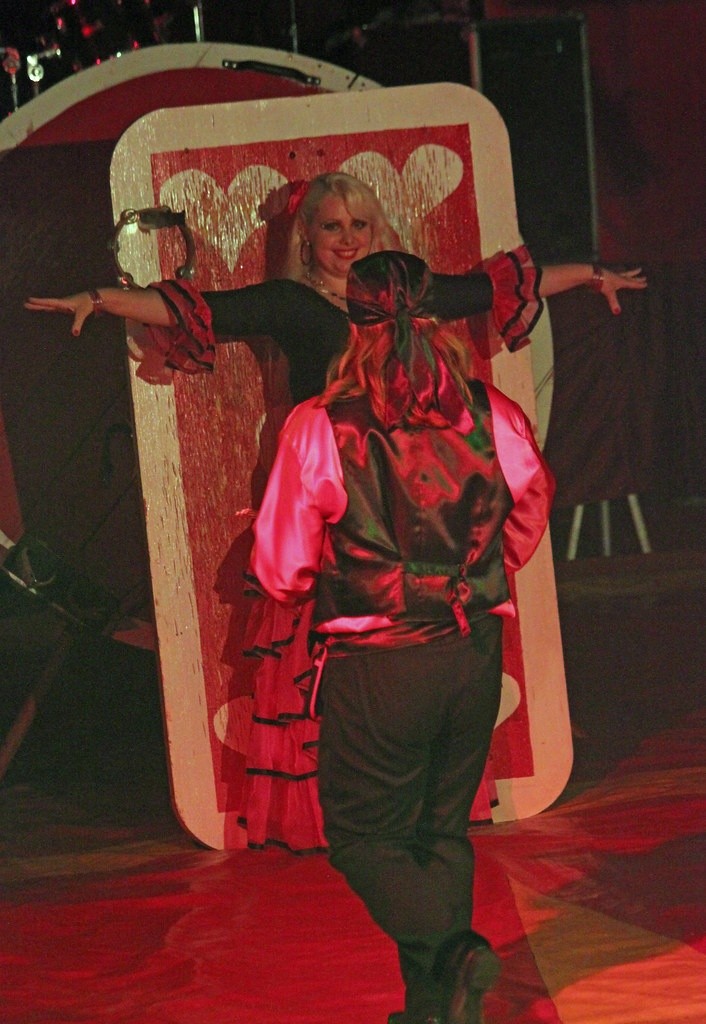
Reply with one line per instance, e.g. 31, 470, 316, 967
624, 270, 627, 277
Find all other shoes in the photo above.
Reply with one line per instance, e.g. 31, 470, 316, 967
387, 1006, 446, 1024
439, 928, 498, 1024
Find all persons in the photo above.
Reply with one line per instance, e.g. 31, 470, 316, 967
25, 172, 647, 850
250, 251, 551, 1024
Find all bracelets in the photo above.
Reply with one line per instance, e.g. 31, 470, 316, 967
592, 263, 604, 293
87, 289, 105, 320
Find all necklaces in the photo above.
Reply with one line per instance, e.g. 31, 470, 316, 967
305, 271, 346, 302
